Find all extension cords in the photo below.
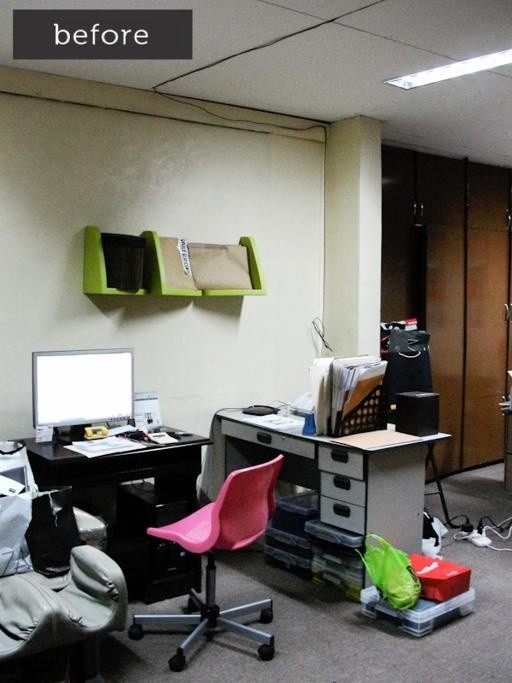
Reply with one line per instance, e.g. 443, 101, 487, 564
459, 528, 491, 548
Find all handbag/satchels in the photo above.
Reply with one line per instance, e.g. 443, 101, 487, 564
0, 486, 81, 577
408, 552, 471, 602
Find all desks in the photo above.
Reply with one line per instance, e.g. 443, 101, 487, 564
16, 425, 216, 606
212, 408, 453, 600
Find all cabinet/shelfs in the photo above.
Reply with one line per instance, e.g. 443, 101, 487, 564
382, 142, 512, 484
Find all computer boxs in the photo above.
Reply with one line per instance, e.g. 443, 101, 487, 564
109, 481, 202, 607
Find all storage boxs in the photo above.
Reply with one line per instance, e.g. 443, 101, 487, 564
261, 489, 365, 600
358, 583, 478, 636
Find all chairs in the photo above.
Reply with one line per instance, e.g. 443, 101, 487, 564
127, 451, 287, 671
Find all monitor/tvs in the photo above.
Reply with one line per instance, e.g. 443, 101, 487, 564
31, 348, 134, 446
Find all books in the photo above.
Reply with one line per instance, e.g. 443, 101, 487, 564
71, 434, 136, 452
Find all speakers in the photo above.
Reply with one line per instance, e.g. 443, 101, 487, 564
394, 391, 439, 437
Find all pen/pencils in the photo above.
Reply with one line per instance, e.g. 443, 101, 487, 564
166, 431, 193, 436
